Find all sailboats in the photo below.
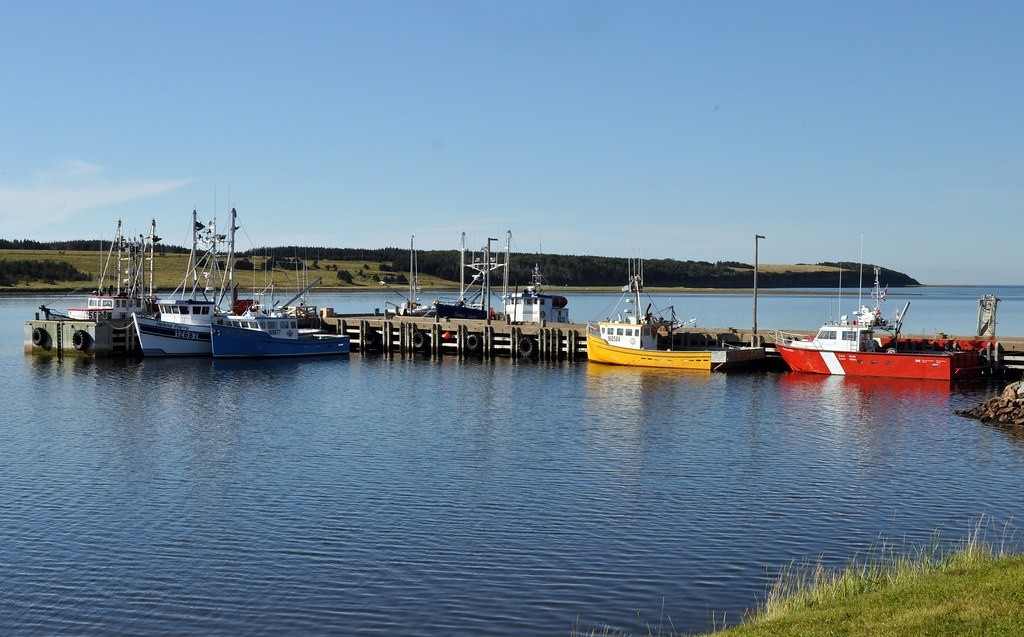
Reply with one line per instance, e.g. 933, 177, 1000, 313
131, 208, 238, 357
209, 208, 351, 359
24, 217, 163, 361
378, 232, 503, 322
154, 210, 230, 326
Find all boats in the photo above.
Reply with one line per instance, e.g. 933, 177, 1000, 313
769, 235, 980, 382
502, 242, 569, 322
585, 238, 767, 371
